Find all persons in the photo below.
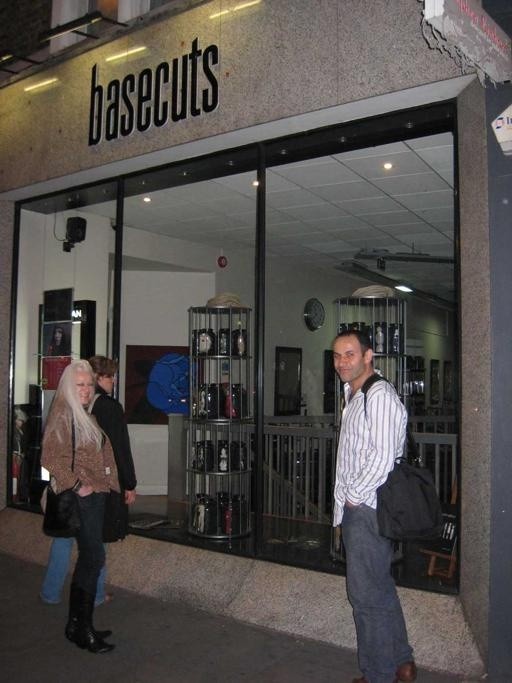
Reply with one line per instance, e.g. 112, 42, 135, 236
208, 361, 248, 418
324, 328, 421, 683
11, 406, 30, 501
37, 358, 125, 656
29, 351, 138, 608
45, 323, 70, 357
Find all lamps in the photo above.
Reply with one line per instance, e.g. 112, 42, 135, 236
377, 257, 385, 273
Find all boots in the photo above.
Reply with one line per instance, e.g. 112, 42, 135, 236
64, 585, 116, 654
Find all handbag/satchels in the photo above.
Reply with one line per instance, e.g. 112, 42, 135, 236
43, 484, 85, 539
375, 461, 446, 543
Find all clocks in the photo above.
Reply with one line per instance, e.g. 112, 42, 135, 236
304, 298, 325, 332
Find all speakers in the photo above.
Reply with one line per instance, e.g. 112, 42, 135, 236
65, 217, 87, 242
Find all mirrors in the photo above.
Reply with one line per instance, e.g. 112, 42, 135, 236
274, 346, 302, 417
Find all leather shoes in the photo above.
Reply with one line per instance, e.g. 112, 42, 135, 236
351, 659, 417, 683
